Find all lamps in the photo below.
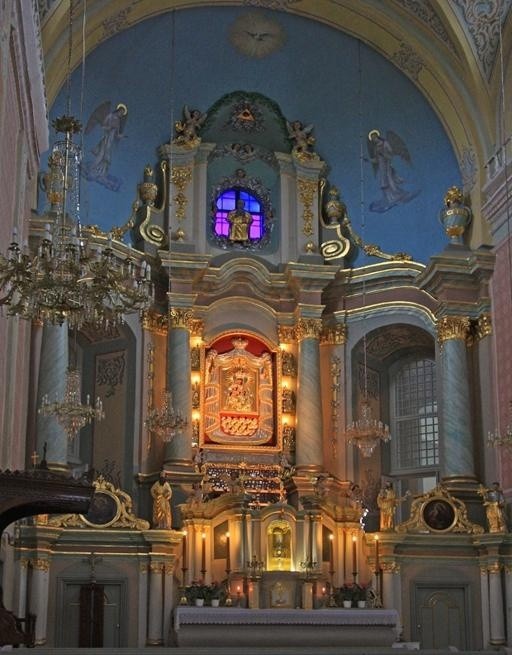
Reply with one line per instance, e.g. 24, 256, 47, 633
0, 0, 512, 457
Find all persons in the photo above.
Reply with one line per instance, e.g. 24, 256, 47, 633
186, 109, 201, 141
188, 480, 204, 509
227, 471, 242, 495
482, 480, 507, 535
315, 475, 331, 497
376, 480, 398, 531
90, 106, 128, 178
227, 377, 254, 412
287, 120, 311, 154
347, 484, 363, 512
149, 470, 175, 529
227, 199, 253, 246
360, 132, 404, 203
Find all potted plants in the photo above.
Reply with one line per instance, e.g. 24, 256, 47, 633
194, 588, 209, 607
352, 589, 367, 608
338, 588, 354, 608
208, 589, 223, 608
